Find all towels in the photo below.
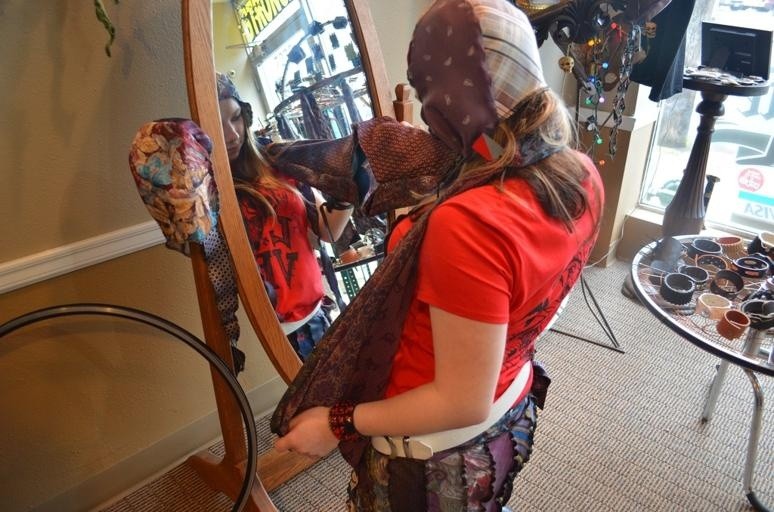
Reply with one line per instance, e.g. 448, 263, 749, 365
300, 88, 336, 141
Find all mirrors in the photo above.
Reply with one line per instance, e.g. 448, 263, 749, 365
177, 0, 412, 512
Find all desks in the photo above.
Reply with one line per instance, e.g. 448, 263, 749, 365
631, 234, 774, 512
621, 68, 772, 303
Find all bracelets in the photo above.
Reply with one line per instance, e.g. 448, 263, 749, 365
337, 241, 387, 265
660, 230, 774, 340
328, 399, 368, 446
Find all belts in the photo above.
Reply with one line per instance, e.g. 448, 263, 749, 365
373, 358, 531, 459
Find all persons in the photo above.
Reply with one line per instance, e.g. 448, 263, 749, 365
270, 0, 605, 512
216, 73, 354, 365
